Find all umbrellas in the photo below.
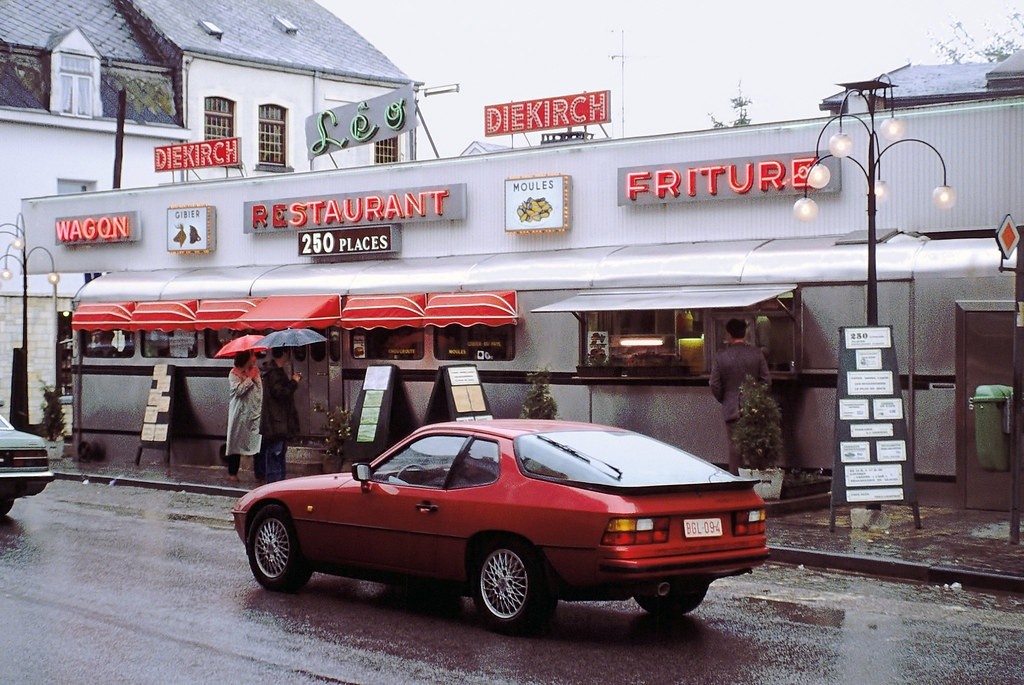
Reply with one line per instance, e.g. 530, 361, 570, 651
214, 334, 272, 368
253, 325, 328, 376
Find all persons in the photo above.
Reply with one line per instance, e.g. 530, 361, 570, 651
711, 317, 771, 477
225, 349, 266, 484
258, 346, 303, 485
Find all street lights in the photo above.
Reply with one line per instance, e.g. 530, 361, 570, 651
793, 73, 954, 522
0, 213, 60, 433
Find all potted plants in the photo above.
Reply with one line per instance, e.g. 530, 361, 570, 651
593, 353, 622, 377
38, 379, 67, 461
623, 352, 690, 377
727, 374, 785, 501
312, 401, 354, 474
576, 354, 594, 377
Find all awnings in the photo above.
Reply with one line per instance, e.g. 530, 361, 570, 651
70, 292, 519, 333
530, 285, 797, 323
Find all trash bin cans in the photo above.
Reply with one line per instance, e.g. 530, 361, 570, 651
972, 384, 1013, 472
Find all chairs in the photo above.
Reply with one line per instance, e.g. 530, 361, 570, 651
425, 447, 493, 488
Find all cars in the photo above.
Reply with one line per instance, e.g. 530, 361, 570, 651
0, 415, 56, 513
232, 420, 769, 633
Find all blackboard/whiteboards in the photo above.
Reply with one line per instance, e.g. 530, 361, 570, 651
140, 364, 177, 447
422, 363, 494, 426
831, 326, 917, 504
348, 364, 398, 457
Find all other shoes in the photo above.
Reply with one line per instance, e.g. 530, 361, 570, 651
229, 475, 239, 482
